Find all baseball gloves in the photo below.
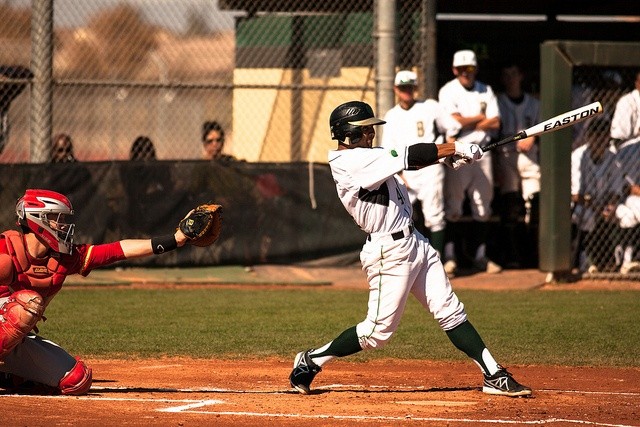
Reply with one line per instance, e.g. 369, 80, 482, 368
176, 203, 223, 247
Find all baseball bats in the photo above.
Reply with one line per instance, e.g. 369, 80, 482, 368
451, 101, 603, 171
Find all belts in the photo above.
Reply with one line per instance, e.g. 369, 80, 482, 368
367, 222, 415, 242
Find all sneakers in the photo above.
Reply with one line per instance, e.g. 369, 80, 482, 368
474, 256, 502, 274
289, 347, 323, 395
482, 368, 532, 397
441, 261, 456, 279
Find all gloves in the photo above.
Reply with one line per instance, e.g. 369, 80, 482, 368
439, 154, 468, 172
455, 141, 485, 165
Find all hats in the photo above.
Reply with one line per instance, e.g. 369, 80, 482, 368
452, 50, 478, 67
394, 70, 418, 88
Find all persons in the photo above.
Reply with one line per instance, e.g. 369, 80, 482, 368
189, 120, 273, 266
570, 117, 639, 274
381, 69, 444, 262
438, 48, 502, 280
1, 187, 222, 396
28, 134, 97, 227
291, 100, 533, 399
493, 59, 539, 218
610, 75, 639, 273
120, 136, 191, 267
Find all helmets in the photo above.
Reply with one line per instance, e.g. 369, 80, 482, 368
329, 101, 387, 145
15, 189, 76, 256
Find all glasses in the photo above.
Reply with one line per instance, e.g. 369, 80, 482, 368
206, 137, 223, 143
56, 147, 71, 153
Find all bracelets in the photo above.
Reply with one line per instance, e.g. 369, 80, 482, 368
151, 234, 179, 255
